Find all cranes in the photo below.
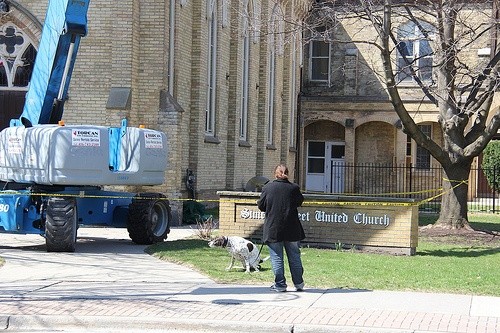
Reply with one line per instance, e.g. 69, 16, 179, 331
0, 0, 173, 251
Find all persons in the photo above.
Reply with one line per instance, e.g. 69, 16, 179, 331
257, 164, 306, 292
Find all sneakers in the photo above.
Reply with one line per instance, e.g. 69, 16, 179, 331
270, 283, 286, 292
294, 281, 305, 291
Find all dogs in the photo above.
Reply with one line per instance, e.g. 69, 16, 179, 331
208, 235, 270, 273
